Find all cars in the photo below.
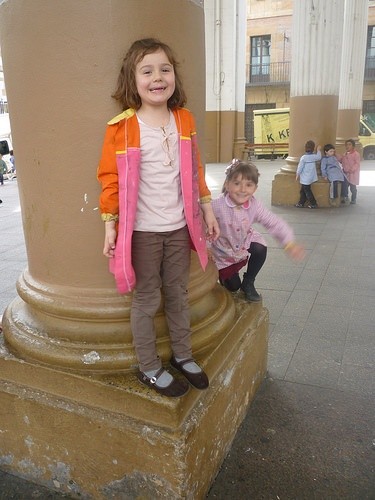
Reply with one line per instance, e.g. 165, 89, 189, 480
1, 138, 15, 174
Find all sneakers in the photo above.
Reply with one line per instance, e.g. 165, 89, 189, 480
351, 200, 356, 204
306, 203, 318, 208
340, 200, 350, 206
294, 202, 304, 207
329, 198, 336, 204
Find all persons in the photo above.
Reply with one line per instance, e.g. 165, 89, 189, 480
296, 141, 322, 208
0, 151, 17, 185
96, 38, 221, 397
340, 139, 360, 204
210, 159, 305, 302
321, 144, 350, 206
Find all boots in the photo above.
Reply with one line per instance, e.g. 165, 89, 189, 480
241, 274, 261, 303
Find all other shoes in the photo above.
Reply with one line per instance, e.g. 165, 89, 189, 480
170, 353, 209, 389
138, 366, 190, 399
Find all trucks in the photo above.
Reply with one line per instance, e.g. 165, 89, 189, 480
252, 108, 375, 161
